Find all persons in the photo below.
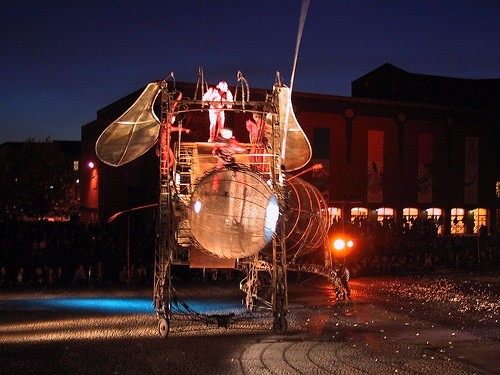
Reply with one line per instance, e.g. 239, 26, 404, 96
160, 93, 191, 176
334, 263, 351, 299
329, 240, 500, 278
2, 210, 230, 284
202, 81, 233, 142
234, 105, 268, 177
